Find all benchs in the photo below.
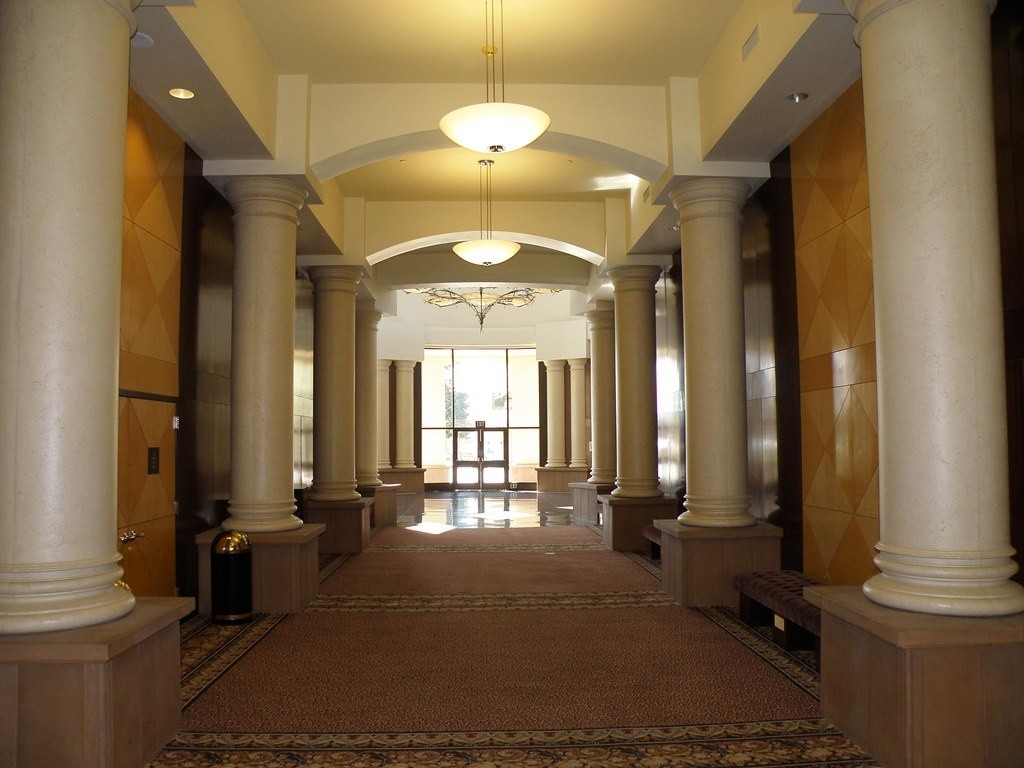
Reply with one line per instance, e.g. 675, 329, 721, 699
596, 504, 603, 524
642, 524, 661, 560
733, 570, 822, 673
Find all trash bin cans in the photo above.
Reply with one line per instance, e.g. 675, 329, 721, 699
211, 529, 253, 624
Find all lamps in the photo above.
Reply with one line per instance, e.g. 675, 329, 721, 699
438, 0, 552, 153
401, 286, 563, 332
451, 160, 520, 267
